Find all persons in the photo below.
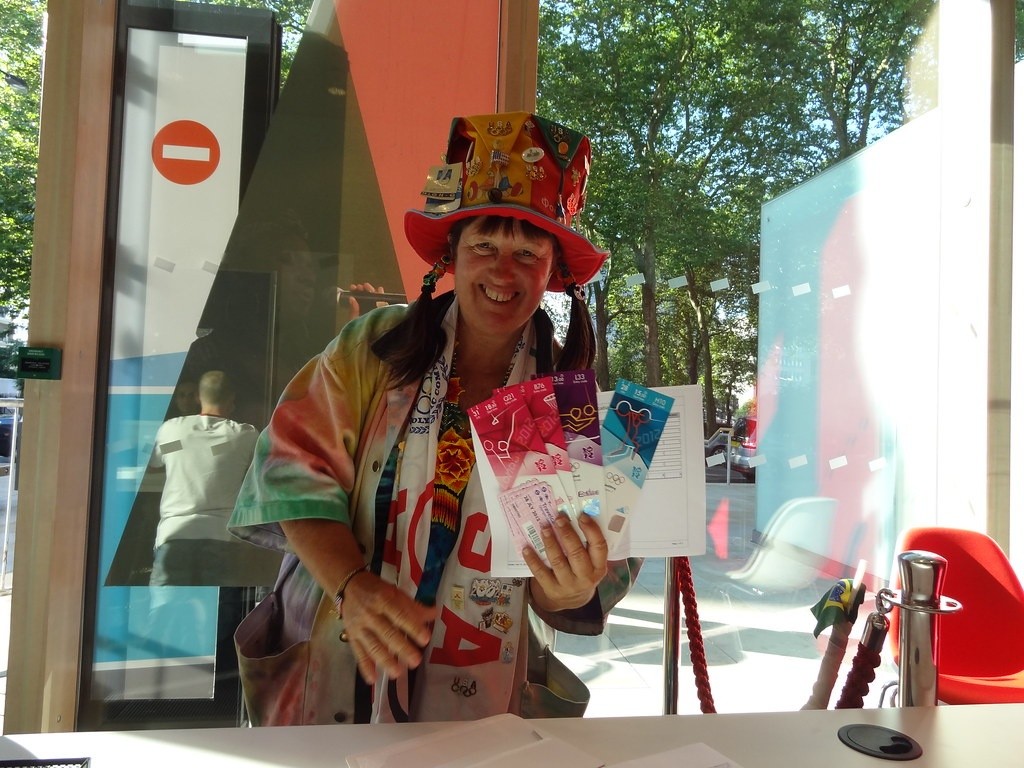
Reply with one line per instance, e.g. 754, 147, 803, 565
147, 371, 263, 585
227, 113, 643, 727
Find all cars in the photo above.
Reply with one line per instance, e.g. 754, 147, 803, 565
704, 427, 732, 466
0, 352, 29, 465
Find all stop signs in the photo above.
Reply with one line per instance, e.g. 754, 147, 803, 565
153, 119, 220, 187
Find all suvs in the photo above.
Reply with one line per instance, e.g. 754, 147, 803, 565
722, 416, 759, 482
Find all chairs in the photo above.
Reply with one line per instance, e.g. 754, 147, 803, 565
875, 524, 1024, 708
701, 496, 838, 658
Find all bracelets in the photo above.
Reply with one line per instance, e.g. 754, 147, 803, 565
330, 566, 370, 618
